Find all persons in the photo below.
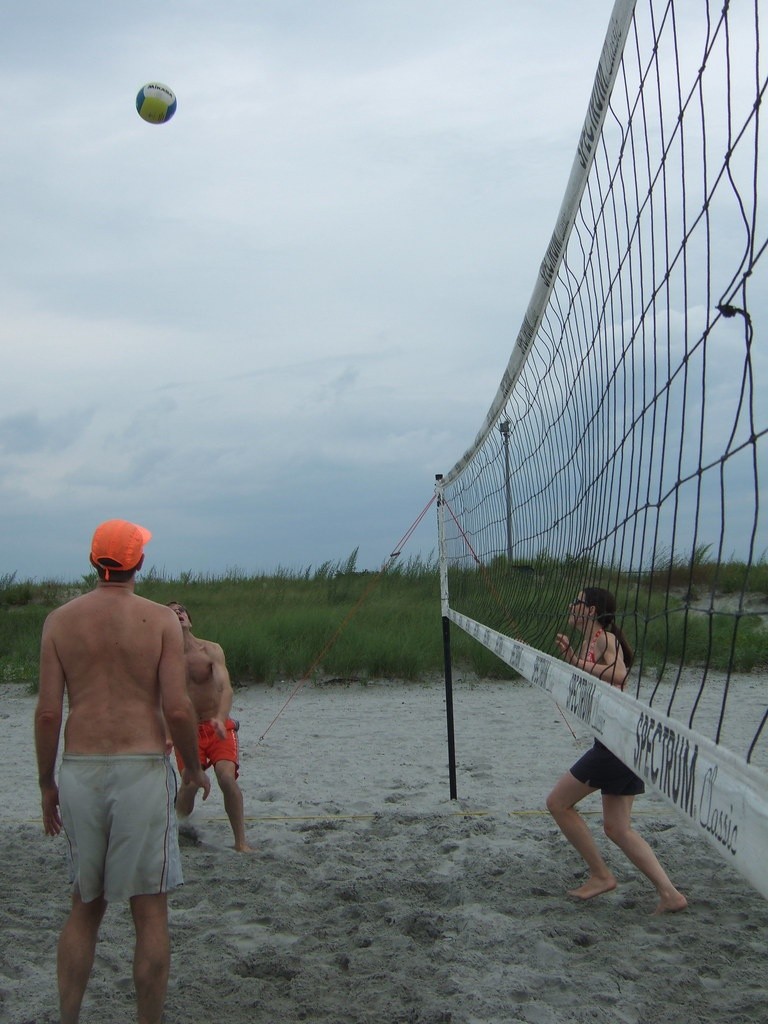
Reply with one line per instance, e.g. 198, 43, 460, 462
546, 587, 688, 917
165, 601, 254, 852
34, 519, 211, 1024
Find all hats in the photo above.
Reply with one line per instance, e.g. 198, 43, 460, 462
91, 519, 152, 581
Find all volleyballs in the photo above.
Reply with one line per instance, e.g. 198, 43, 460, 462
135, 82, 178, 126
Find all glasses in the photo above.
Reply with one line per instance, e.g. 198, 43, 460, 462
571, 598, 586, 606
173, 607, 186, 613
